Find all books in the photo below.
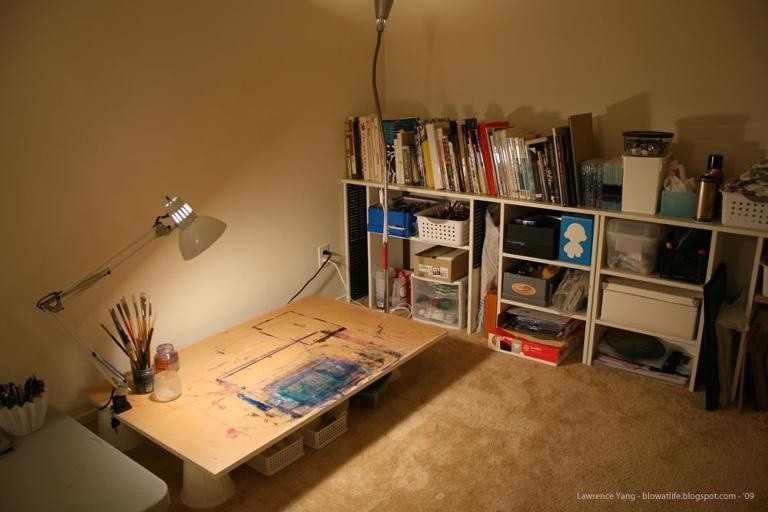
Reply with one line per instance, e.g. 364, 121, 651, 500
592, 327, 694, 388
714, 300, 734, 406
344, 112, 624, 212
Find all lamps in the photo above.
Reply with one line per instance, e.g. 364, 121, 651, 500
369, 1, 396, 313
37, 191, 228, 414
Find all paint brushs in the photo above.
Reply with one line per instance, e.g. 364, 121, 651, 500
99, 289, 153, 389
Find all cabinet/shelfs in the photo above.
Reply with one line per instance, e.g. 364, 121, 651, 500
342, 177, 768, 404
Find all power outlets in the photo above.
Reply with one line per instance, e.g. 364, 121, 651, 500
317, 242, 331, 269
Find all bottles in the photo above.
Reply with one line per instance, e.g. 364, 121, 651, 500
155, 344, 179, 374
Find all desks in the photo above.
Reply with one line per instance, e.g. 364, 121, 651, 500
81, 292, 448, 509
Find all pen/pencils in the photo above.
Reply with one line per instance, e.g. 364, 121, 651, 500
1, 374, 49, 413
425, 200, 473, 227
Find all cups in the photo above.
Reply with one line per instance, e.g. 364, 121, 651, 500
154, 371, 182, 401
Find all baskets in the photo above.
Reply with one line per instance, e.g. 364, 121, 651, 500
301, 413, 348, 448
718, 187, 768, 232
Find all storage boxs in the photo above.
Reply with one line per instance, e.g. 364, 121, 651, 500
662, 191, 700, 218
606, 219, 663, 276
302, 406, 350, 449
600, 277, 701, 341
622, 129, 675, 156
412, 246, 468, 282
350, 371, 393, 410
621, 151, 674, 215
560, 215, 594, 266
504, 217, 558, 260
502, 262, 562, 308
719, 180, 768, 230
366, 195, 440, 238
